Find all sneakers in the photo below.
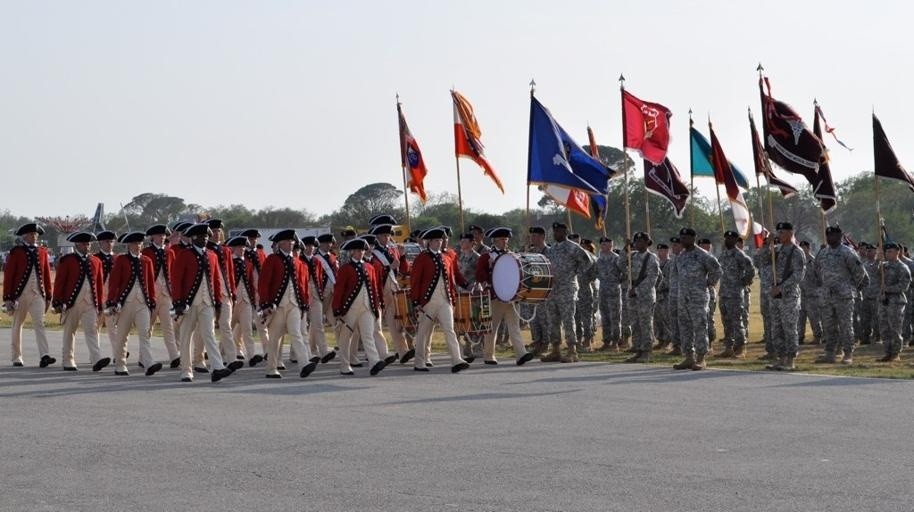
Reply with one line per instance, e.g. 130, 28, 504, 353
321, 348, 533, 376
11, 352, 132, 381
138, 351, 321, 382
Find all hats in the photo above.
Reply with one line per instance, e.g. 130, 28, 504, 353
529, 222, 613, 244
67, 231, 145, 243
633, 228, 738, 249
777, 222, 792, 230
410, 225, 513, 239
800, 226, 909, 250
269, 230, 336, 250
146, 220, 224, 237
15, 224, 45, 235
339, 214, 397, 249
225, 229, 261, 247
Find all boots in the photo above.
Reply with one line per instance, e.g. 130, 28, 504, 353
841, 351, 854, 364
541, 338, 619, 362
720, 346, 795, 370
627, 339, 713, 369
857, 338, 911, 362
816, 348, 836, 364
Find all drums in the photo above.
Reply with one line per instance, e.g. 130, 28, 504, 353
392, 288, 420, 333
490, 252, 554, 304
454, 289, 493, 336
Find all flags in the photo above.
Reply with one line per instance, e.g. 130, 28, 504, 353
690, 125, 747, 184
708, 123, 750, 241
545, 182, 589, 217
763, 91, 838, 216
644, 153, 690, 218
398, 99, 428, 204
748, 116, 797, 199
587, 128, 606, 230
872, 115, 914, 193
622, 90, 672, 165
752, 221, 770, 248
528, 92, 611, 229
812, 104, 823, 140
452, 91, 504, 195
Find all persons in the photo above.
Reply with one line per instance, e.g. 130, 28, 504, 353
1, 219, 913, 388
